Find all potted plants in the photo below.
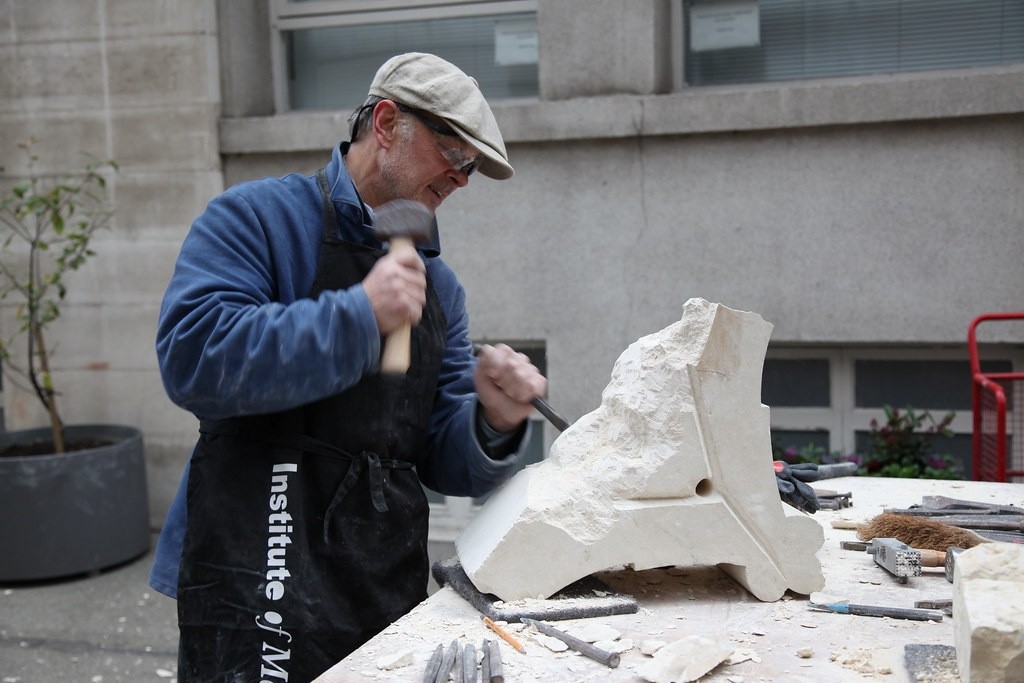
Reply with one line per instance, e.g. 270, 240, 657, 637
0, 133, 157, 590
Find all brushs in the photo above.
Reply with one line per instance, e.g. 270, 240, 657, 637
857, 512, 1015, 555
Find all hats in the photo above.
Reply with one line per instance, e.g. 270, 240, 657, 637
369, 51, 514, 181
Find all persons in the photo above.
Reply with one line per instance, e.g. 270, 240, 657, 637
150, 50, 546, 683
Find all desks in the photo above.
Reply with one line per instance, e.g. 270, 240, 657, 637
297, 476, 1024, 677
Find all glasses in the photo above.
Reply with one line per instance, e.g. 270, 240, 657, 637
397, 104, 484, 177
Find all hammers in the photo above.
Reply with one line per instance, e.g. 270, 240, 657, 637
369, 198, 431, 375
866, 537, 947, 579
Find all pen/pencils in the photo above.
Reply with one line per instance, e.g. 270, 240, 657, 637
479, 613, 530, 657
